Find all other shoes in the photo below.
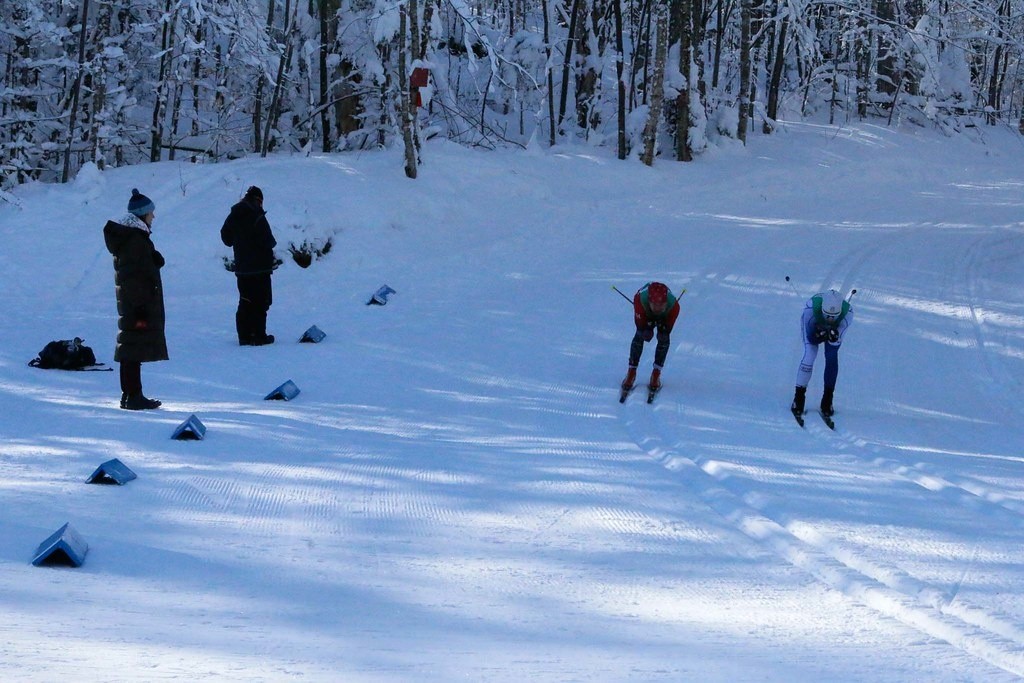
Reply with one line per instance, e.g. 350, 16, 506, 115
244, 335, 275, 346
120, 391, 162, 410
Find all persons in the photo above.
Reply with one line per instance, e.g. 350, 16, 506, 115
791, 290, 854, 416
220, 186, 277, 345
103, 188, 169, 410
622, 282, 679, 389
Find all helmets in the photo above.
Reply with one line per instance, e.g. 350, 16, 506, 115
648, 282, 667, 304
821, 291, 843, 316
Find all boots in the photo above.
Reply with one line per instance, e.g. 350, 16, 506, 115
649, 368, 661, 389
792, 384, 807, 415
622, 367, 637, 390
820, 391, 835, 417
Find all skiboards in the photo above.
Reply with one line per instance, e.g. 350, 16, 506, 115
616, 376, 661, 404
790, 406, 839, 431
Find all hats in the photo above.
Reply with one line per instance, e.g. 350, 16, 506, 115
127, 188, 154, 216
247, 186, 263, 202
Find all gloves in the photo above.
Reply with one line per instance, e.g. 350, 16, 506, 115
658, 330, 666, 341
828, 329, 840, 342
811, 328, 828, 344
642, 326, 655, 342
